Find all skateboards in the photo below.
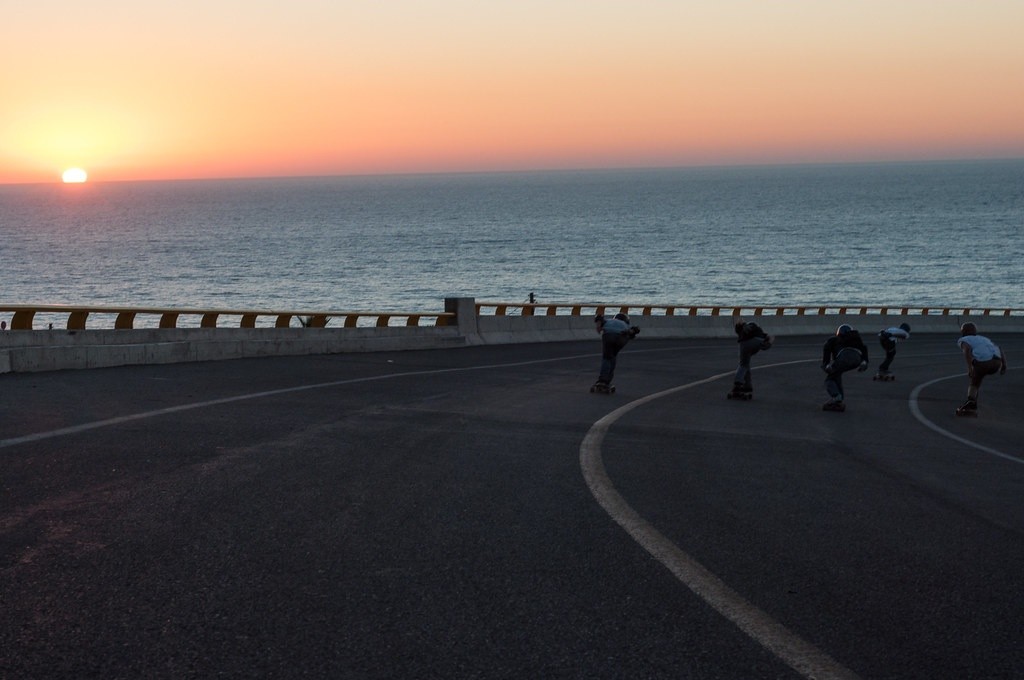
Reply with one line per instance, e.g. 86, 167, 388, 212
956, 405, 977, 417
823, 400, 845, 412
590, 381, 615, 393
873, 372, 895, 381
728, 387, 752, 400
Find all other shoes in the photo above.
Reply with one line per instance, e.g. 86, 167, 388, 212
735, 384, 753, 394
829, 394, 844, 403
879, 370, 892, 375
962, 399, 978, 411
595, 378, 612, 388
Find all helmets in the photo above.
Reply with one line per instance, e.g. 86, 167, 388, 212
836, 324, 853, 336
961, 322, 977, 333
615, 313, 630, 324
900, 323, 910, 331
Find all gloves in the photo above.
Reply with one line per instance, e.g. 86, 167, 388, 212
742, 322, 752, 334
880, 330, 891, 338
821, 364, 831, 373
858, 359, 869, 371
760, 342, 771, 350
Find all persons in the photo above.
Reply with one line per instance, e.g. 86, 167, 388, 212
727, 320, 775, 399
590, 311, 640, 393
875, 323, 911, 380
954, 322, 1006, 415
822, 324, 869, 411
